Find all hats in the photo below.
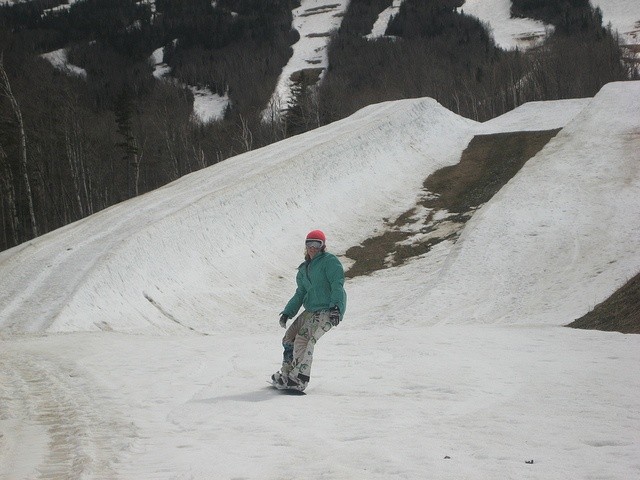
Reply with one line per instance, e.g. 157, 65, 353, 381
307, 230, 325, 240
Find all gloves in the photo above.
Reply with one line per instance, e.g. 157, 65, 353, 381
279, 314, 288, 329
329, 306, 340, 326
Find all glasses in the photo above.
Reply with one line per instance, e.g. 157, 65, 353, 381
306, 239, 323, 248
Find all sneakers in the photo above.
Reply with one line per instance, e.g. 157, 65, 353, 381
272, 371, 282, 380
278, 377, 300, 386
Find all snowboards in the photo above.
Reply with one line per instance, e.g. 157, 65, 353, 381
272, 373, 306, 395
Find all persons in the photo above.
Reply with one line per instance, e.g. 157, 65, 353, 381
272, 229, 347, 391
